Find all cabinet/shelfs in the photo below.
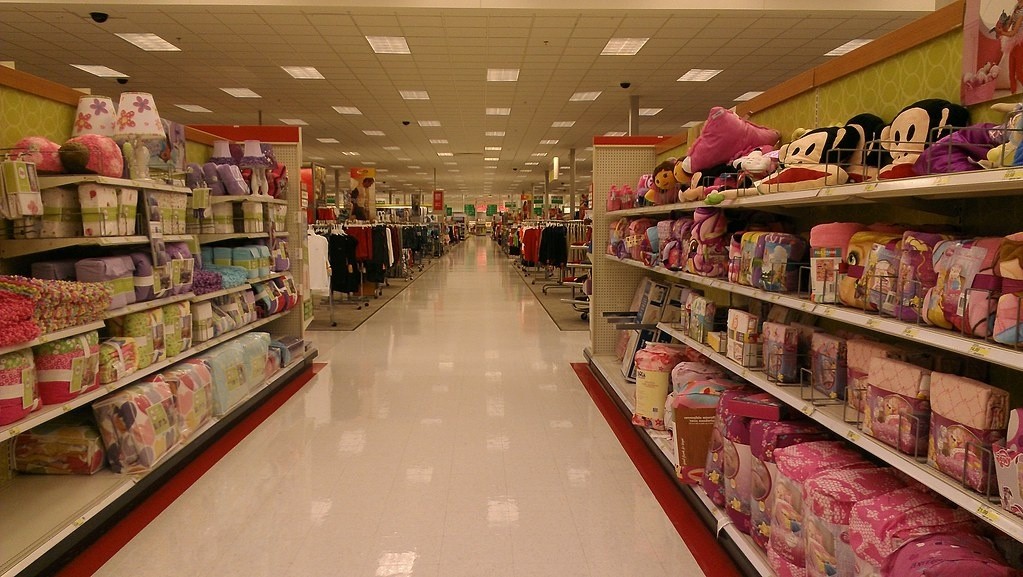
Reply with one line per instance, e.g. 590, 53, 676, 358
601, 164, 1023, 577
560, 244, 593, 305
0, 173, 305, 577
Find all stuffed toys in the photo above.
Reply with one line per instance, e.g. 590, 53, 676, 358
634, 97, 1023, 207
606, 210, 1023, 350
838, 231, 902, 311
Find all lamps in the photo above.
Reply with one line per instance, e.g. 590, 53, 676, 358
208, 141, 244, 172
71, 95, 117, 138
113, 92, 167, 183
239, 139, 278, 199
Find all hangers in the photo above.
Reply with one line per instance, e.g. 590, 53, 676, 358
520, 217, 593, 233
307, 221, 428, 238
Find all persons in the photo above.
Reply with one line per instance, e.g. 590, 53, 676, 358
996, 0, 1023, 94
